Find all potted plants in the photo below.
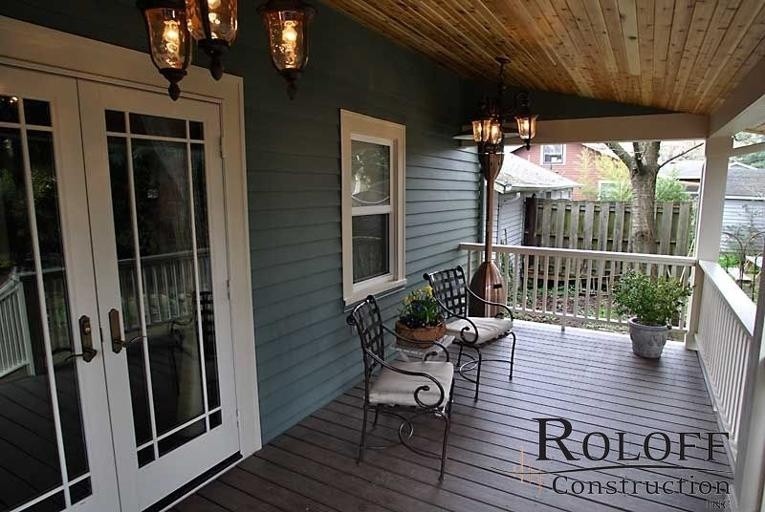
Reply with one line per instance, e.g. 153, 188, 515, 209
611, 268, 699, 359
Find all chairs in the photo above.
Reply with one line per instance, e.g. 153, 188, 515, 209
347, 265, 515, 481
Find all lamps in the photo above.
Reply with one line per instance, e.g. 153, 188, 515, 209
470, 55, 540, 149
140, 0, 310, 100
